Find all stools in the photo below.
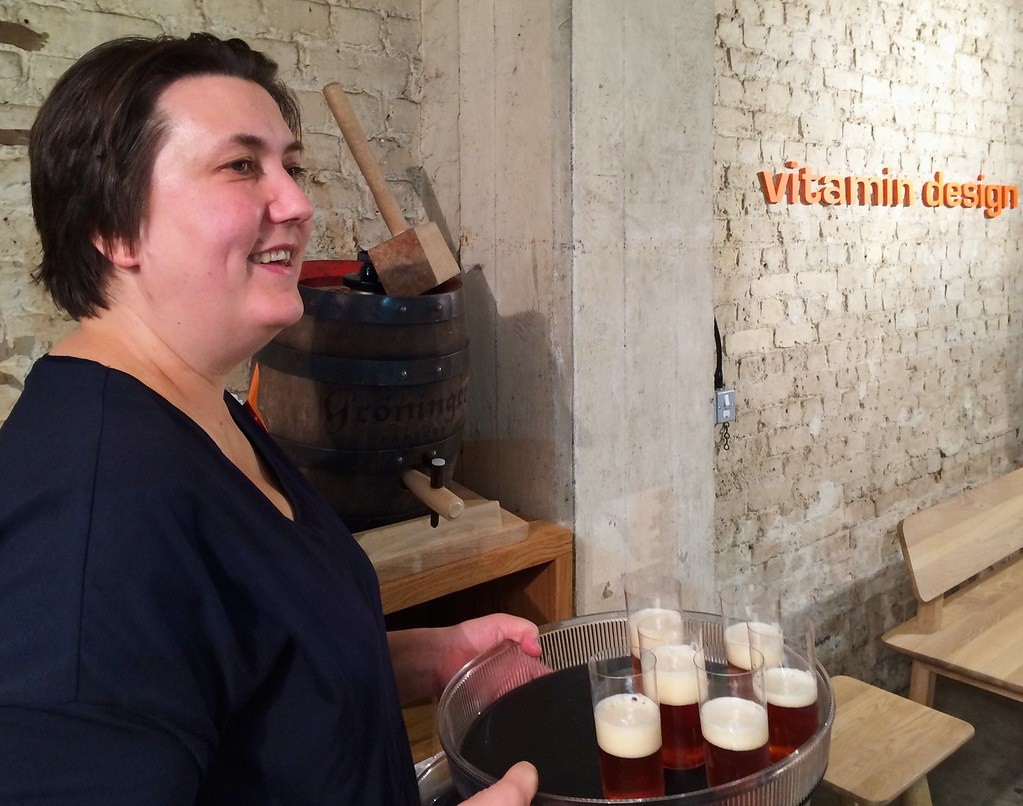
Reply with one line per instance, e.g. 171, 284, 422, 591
820, 674, 976, 806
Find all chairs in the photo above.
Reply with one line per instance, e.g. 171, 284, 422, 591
879, 466, 1022, 708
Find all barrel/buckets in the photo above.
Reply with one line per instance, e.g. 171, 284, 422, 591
249, 259, 470, 535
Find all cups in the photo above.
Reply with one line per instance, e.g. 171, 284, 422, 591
588, 576, 816, 758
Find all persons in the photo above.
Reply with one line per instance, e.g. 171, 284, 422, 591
1, 33, 554, 806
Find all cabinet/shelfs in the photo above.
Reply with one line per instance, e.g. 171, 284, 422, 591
378, 507, 574, 764
